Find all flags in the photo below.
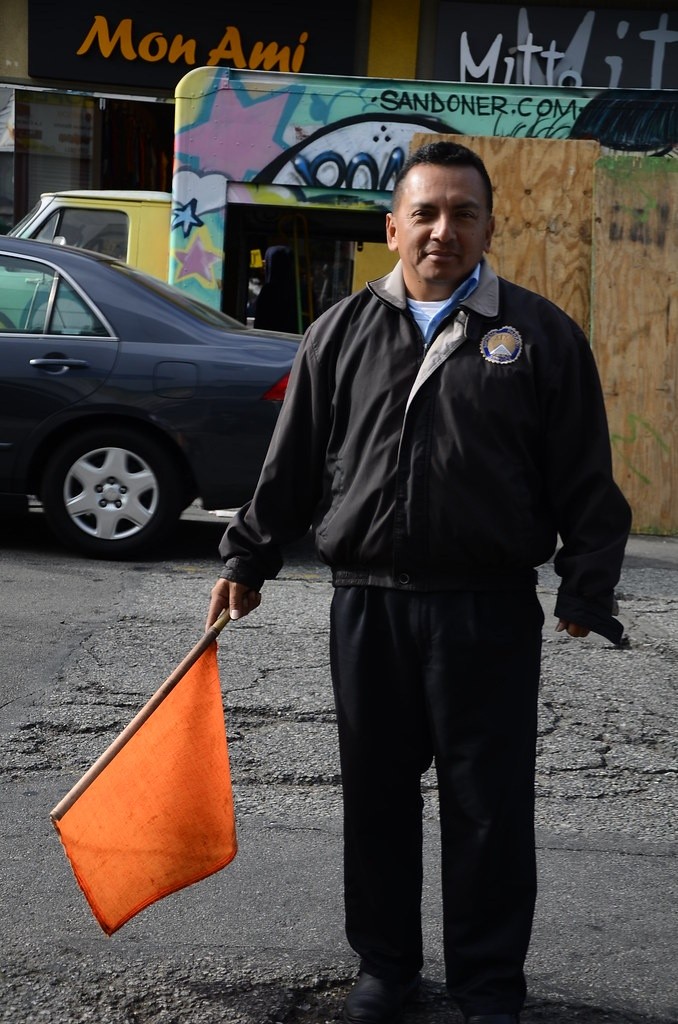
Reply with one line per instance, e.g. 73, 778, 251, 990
53, 640, 239, 938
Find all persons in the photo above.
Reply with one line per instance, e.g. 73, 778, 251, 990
208, 141, 632, 1024
243, 244, 321, 334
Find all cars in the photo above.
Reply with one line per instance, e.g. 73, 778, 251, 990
1, 236, 307, 562
0, 190, 172, 334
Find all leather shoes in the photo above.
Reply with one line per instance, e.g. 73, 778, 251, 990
463, 1013, 520, 1024
345, 968, 422, 1024
